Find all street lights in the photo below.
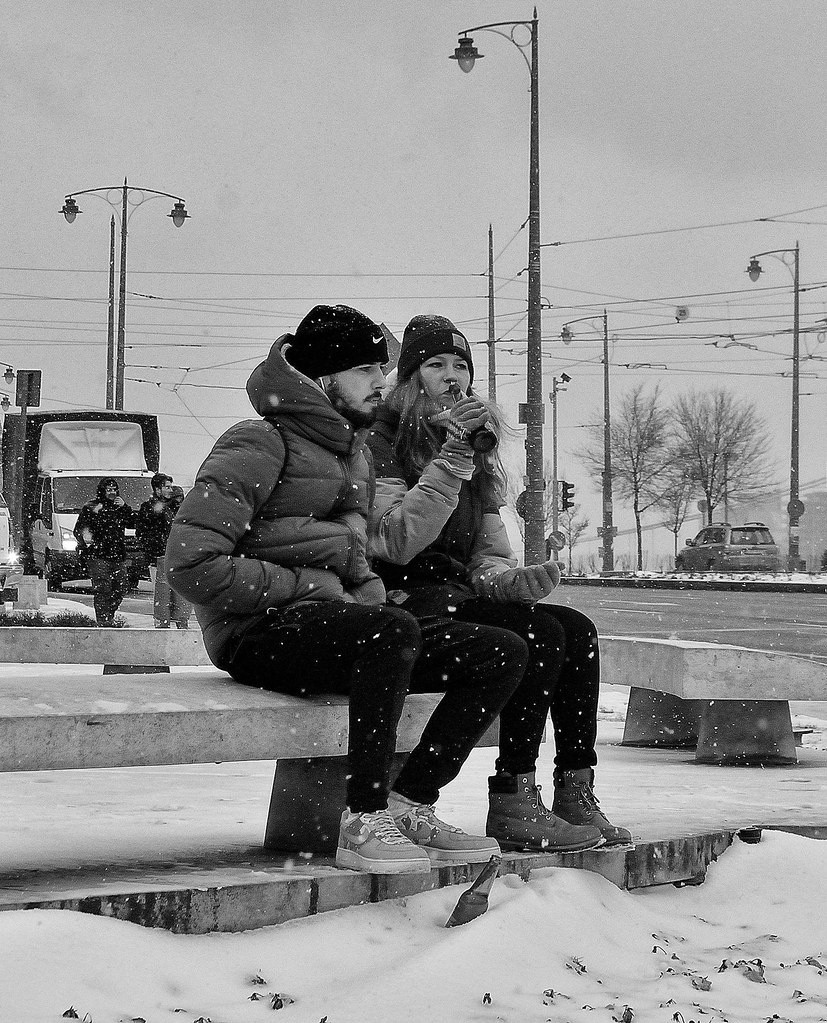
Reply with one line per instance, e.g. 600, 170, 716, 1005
547, 372, 574, 562
447, 8, 547, 576
54, 175, 189, 409
558, 307, 619, 574
742, 241, 809, 572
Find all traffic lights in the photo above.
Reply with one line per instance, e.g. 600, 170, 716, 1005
560, 480, 575, 510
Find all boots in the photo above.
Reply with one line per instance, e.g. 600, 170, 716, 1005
484, 776, 606, 851
552, 769, 631, 844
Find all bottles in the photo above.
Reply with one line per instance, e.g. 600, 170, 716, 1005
449, 383, 497, 453
445, 855, 501, 927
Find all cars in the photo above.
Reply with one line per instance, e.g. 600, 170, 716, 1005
0, 495, 23, 584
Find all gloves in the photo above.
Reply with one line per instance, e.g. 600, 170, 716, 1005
489, 561, 565, 603
440, 395, 490, 469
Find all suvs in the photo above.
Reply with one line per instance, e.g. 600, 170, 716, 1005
672, 519, 780, 570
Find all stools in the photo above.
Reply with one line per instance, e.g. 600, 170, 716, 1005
12, 583, 40, 610
20, 579, 47, 605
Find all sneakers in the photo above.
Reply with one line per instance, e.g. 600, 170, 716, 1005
389, 804, 503, 862
336, 806, 432, 872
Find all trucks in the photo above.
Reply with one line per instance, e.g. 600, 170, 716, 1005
3, 411, 164, 589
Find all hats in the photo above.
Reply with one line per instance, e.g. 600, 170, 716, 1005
399, 315, 474, 397
294, 305, 388, 382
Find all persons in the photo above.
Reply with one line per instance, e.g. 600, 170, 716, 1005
170, 485, 185, 504
370, 314, 634, 853
714, 532, 723, 543
73, 478, 138, 627
166, 305, 529, 873
136, 472, 192, 629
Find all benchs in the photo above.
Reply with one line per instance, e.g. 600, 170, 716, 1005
598, 636, 827, 766
0, 626, 213, 675
0, 669, 547, 855
